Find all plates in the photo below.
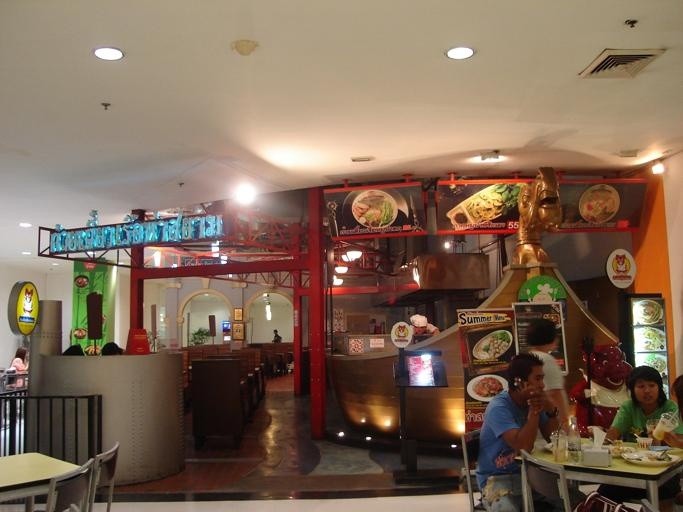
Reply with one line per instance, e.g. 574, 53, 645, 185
443, 182, 506, 232
619, 451, 679, 467
350, 189, 398, 231
470, 329, 513, 361
576, 184, 621, 225
631, 299, 664, 326
465, 373, 509, 403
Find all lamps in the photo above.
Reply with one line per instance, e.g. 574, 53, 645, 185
481, 150, 499, 160
265, 285, 273, 321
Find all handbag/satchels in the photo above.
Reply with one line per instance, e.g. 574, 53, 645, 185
573, 491, 637, 511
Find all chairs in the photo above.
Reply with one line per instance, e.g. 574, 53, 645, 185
31, 457, 96, 511
461, 427, 683, 511
91, 442, 119, 511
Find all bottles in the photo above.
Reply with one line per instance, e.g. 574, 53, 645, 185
567, 417, 582, 466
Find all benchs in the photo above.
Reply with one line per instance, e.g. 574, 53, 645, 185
184, 348, 296, 449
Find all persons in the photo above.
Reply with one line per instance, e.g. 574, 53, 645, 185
648, 374, 683, 511
594, 365, 683, 504
271, 329, 281, 342
473, 350, 562, 511
523, 317, 571, 435
7, 348, 30, 389
407, 313, 440, 345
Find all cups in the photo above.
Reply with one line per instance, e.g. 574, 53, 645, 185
645, 412, 679, 447
549, 430, 567, 463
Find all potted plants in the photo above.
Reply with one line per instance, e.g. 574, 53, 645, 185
192, 329, 211, 344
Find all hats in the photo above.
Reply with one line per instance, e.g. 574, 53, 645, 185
410, 314, 427, 327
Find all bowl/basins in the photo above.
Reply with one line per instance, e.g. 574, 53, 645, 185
634, 438, 653, 449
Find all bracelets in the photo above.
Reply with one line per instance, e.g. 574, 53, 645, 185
546, 406, 558, 417
